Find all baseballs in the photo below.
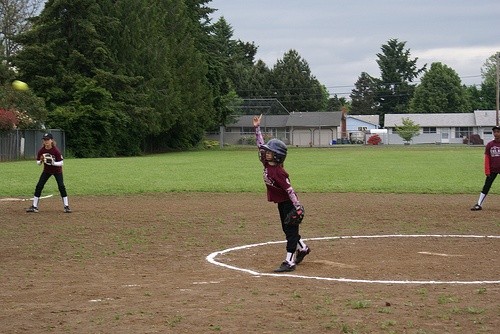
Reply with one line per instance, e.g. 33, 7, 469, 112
13, 80, 28, 92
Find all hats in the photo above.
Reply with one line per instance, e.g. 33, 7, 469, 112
492, 126, 500, 131
42, 132, 54, 140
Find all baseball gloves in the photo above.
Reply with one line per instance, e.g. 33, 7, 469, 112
41, 153, 55, 166
283, 205, 305, 226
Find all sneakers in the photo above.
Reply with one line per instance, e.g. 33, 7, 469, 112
274, 260, 296, 272
63, 206, 72, 213
26, 205, 38, 213
470, 204, 482, 210
295, 245, 311, 264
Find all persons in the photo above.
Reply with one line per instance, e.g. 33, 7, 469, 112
26, 132, 72, 213
470, 126, 500, 211
252, 114, 312, 274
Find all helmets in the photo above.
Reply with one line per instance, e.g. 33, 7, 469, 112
258, 138, 288, 163
